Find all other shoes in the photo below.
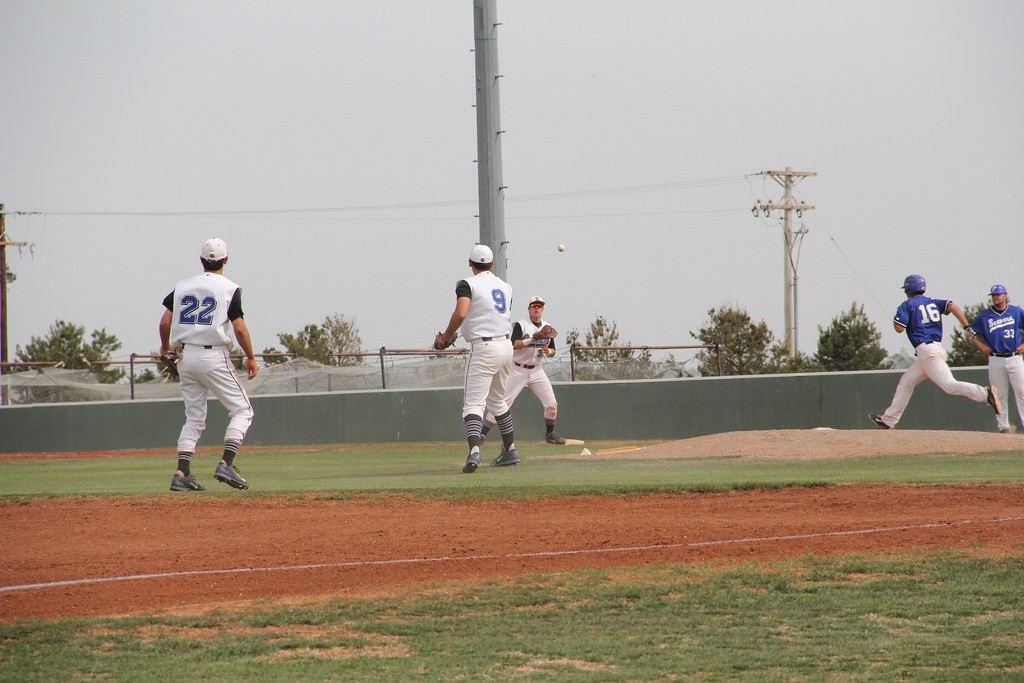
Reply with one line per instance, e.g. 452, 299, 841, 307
987, 384, 1003, 415
868, 412, 891, 430
479, 433, 486, 439
1000, 427, 1009, 433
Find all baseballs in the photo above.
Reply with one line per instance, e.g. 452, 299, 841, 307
558, 244, 565, 252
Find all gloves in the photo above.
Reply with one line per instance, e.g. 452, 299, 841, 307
963, 326, 976, 344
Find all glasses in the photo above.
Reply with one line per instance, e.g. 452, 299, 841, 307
530, 304, 542, 309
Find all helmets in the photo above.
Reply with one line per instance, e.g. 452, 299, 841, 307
470, 244, 494, 264
201, 237, 228, 262
527, 296, 546, 308
987, 284, 1008, 296
900, 274, 927, 297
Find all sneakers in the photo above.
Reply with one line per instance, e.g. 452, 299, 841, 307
214, 461, 249, 490
544, 431, 566, 444
169, 472, 208, 492
462, 452, 481, 473
489, 448, 521, 467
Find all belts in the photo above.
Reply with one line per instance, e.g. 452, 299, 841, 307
514, 362, 535, 370
180, 342, 213, 350
914, 340, 939, 348
988, 351, 1021, 358
483, 334, 510, 341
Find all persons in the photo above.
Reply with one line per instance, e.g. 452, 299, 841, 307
478, 296, 567, 445
435, 244, 521, 474
970, 284, 1024, 434
158, 236, 260, 493
867, 274, 1002, 431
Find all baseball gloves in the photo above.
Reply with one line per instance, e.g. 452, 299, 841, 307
532, 325, 559, 340
434, 331, 458, 350
162, 350, 180, 383
